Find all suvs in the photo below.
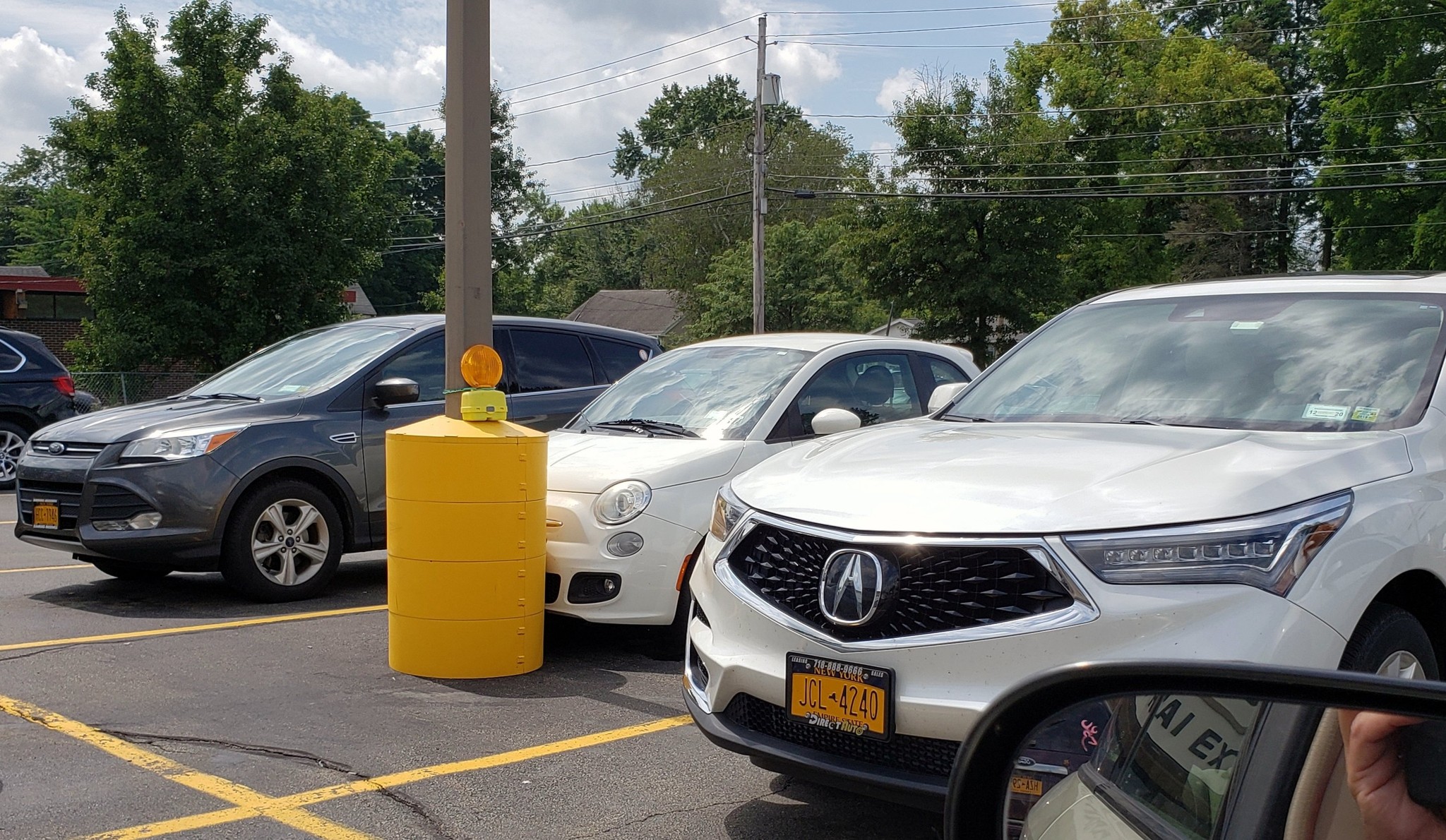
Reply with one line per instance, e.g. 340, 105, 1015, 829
682, 267, 1446, 797
0, 326, 76, 490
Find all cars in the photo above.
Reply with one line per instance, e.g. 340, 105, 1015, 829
544, 333, 981, 626
15, 314, 664, 604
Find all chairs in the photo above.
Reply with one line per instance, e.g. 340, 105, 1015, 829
851, 365, 896, 411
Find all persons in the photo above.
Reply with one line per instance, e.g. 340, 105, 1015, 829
1336, 709, 1446, 840
